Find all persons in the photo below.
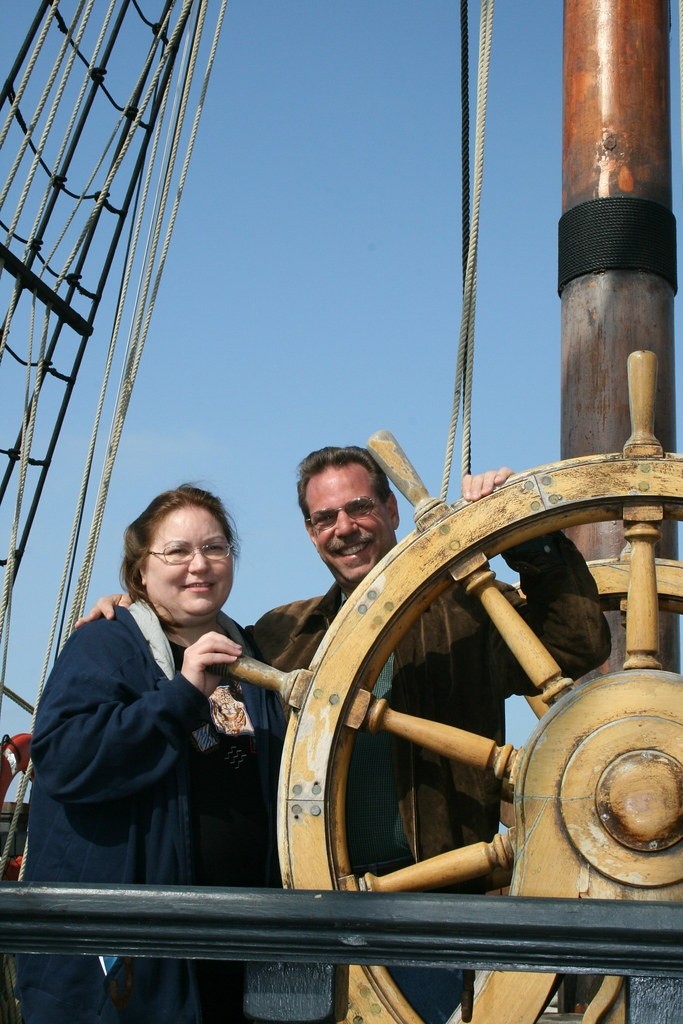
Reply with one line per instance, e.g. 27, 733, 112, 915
73, 447, 611, 1023
17, 485, 289, 1023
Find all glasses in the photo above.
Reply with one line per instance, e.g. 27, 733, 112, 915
148, 542, 234, 565
305, 494, 379, 530
